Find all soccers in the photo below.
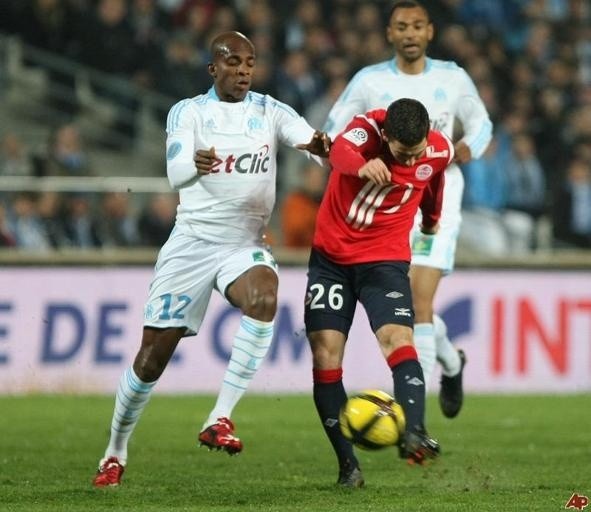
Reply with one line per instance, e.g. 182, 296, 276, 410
339, 391, 406, 451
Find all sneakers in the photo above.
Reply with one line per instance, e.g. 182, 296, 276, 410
92, 456, 124, 490
199, 415, 244, 457
399, 424, 441, 467
336, 466, 365, 492
439, 349, 466, 419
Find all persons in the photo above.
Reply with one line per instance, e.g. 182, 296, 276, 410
300, 95, 459, 495
307, 2, 498, 423
86, 28, 333, 492
